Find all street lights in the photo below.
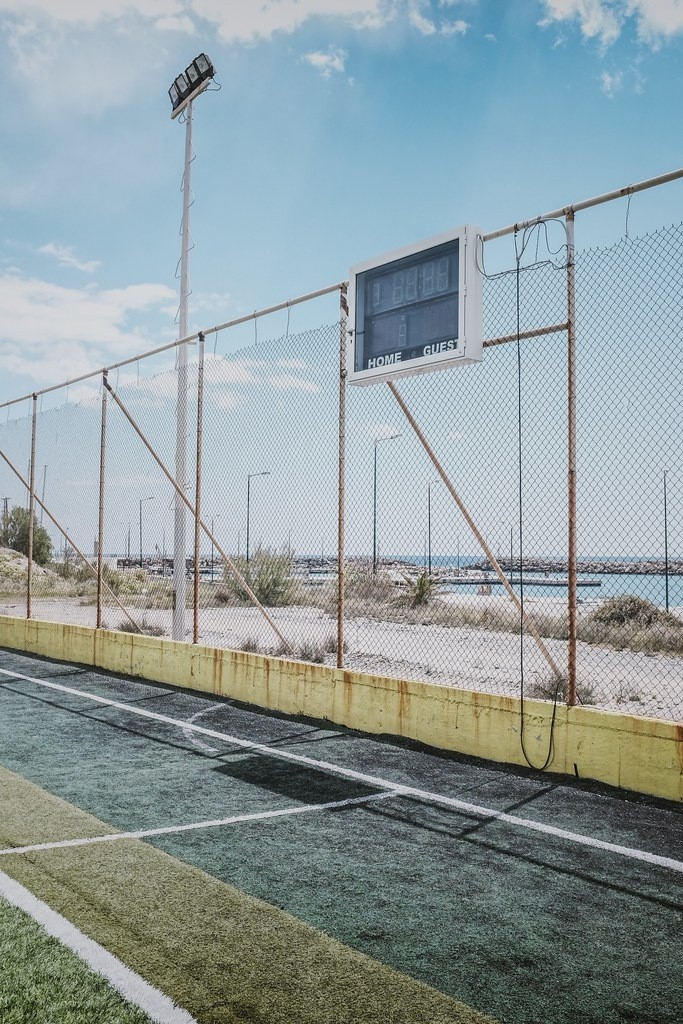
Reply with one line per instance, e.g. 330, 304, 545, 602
139, 496, 154, 569
246, 471, 271, 563
165, 52, 219, 642
373, 433, 403, 575
500, 521, 522, 589
663, 468, 670, 615
203, 514, 220, 583
428, 479, 441, 575
